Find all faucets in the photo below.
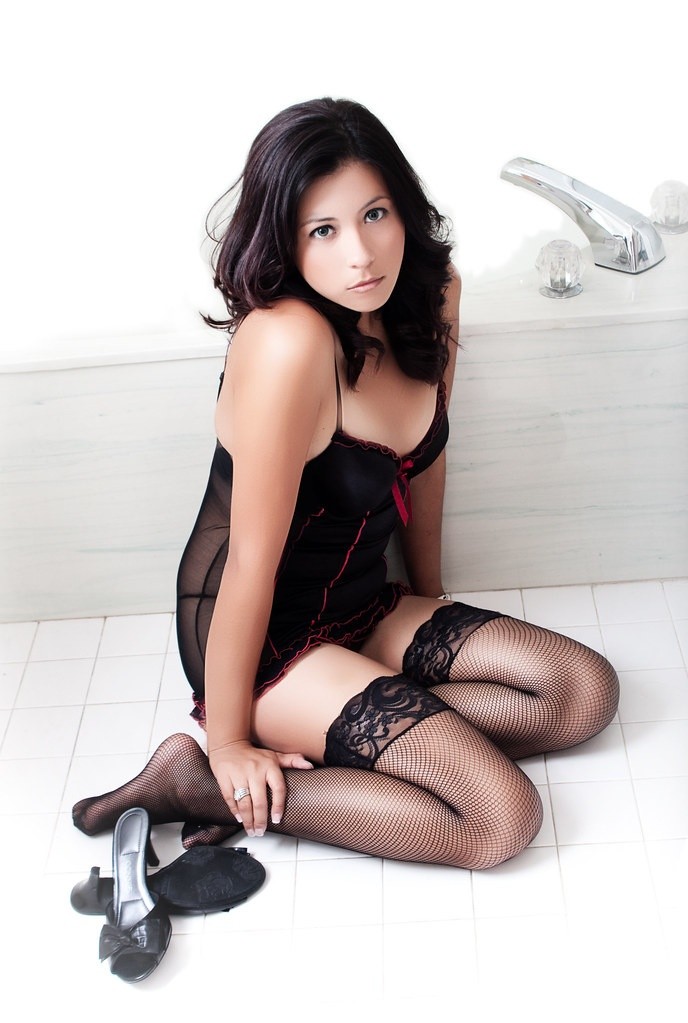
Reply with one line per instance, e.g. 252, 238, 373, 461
500, 155, 668, 276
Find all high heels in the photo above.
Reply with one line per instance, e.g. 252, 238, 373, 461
99, 809, 172, 983
70, 845, 266, 916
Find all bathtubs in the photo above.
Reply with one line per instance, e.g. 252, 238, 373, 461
0, 179, 688, 620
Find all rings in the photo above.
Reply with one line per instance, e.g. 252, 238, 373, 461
234, 788, 250, 802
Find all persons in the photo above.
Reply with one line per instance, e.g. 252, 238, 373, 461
71, 100, 617, 871
